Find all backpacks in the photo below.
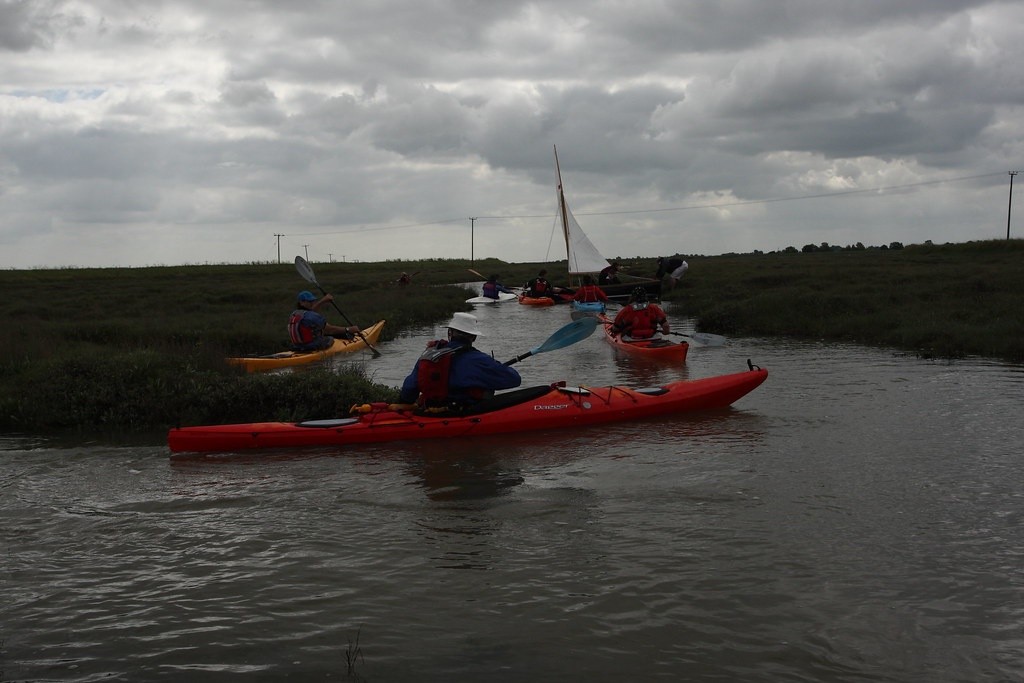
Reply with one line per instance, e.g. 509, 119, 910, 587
417, 341, 463, 399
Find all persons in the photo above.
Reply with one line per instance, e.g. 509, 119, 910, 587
598, 259, 629, 301
482, 275, 514, 298
398, 271, 411, 286
394, 312, 522, 415
610, 285, 670, 339
288, 290, 360, 350
560, 275, 611, 303
654, 256, 688, 304
523, 269, 555, 297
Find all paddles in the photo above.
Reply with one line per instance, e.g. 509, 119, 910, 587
390, 272, 420, 284
466, 268, 520, 298
569, 309, 727, 347
502, 316, 597, 366
294, 255, 382, 357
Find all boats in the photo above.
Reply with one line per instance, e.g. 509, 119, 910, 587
570, 299, 607, 322
518, 293, 554, 306
168, 367, 767, 452
466, 292, 516, 307
222, 320, 388, 372
604, 315, 689, 363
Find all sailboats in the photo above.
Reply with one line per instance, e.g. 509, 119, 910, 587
547, 145, 662, 298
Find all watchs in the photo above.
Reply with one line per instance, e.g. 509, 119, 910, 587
344, 325, 350, 334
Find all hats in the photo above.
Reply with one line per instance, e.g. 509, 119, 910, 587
440, 312, 486, 336
297, 291, 317, 301
